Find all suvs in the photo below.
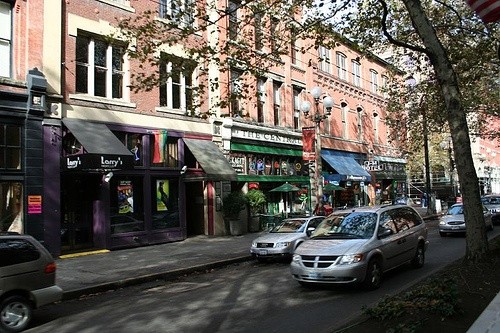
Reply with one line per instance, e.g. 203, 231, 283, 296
290, 202, 429, 292
0, 231, 63, 333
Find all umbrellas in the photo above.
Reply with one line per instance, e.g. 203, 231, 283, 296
311, 183, 347, 208
268, 182, 307, 218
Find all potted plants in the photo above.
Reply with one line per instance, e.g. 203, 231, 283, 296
223, 191, 247, 236
245, 190, 268, 232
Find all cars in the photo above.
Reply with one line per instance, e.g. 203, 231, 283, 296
482, 195, 500, 221
439, 203, 494, 236
250, 215, 328, 262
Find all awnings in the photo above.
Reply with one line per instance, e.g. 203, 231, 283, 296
182, 137, 237, 174
62, 118, 133, 155
320, 154, 371, 176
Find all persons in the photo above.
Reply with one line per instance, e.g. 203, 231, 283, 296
312, 196, 327, 216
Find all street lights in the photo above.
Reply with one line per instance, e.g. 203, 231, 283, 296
440, 136, 457, 201
300, 87, 334, 213
